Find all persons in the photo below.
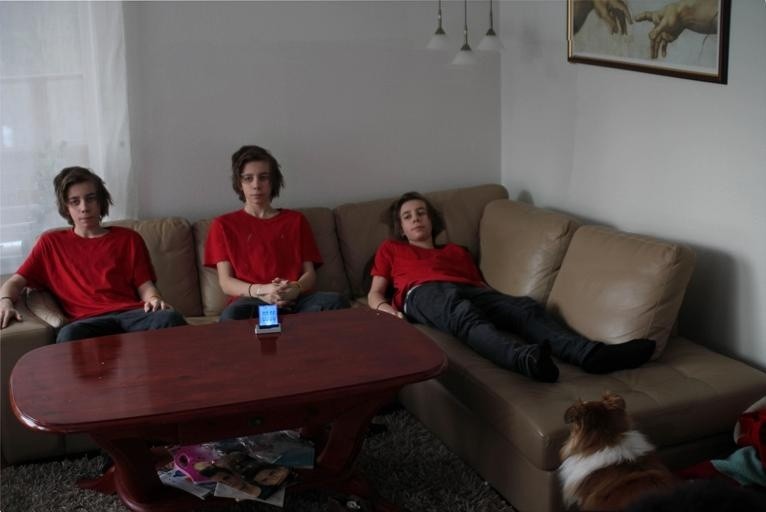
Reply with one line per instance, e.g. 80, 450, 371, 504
200, 145, 350, 324
574, 0, 719, 60
0, 165, 189, 477
366, 192, 658, 384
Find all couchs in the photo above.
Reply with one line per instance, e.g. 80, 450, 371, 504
0, 179, 764, 511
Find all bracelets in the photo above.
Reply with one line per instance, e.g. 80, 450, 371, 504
292, 280, 305, 293
1, 295, 15, 306
376, 301, 387, 310
248, 283, 255, 298
145, 293, 163, 299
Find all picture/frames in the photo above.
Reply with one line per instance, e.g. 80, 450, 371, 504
564, 0, 731, 85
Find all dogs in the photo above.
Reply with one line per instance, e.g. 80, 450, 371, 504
552, 391, 686, 512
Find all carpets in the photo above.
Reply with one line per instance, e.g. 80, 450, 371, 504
0, 410, 520, 512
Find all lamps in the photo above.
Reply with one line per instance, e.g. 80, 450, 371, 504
447, 1, 484, 66
426, 0, 456, 53
473, 0, 506, 52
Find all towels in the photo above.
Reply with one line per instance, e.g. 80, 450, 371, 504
709, 446, 766, 488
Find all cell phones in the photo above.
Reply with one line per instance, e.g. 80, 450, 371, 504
258, 303, 279, 329
